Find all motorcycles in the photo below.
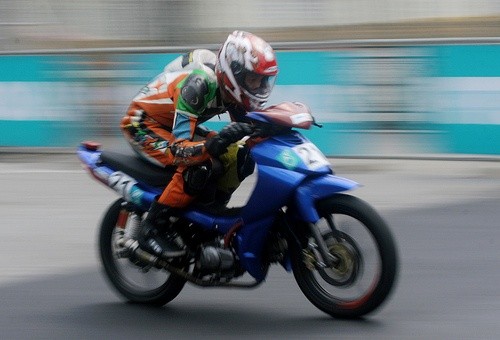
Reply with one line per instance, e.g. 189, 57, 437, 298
76, 98, 399, 321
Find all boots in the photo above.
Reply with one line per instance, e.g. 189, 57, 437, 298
136, 193, 174, 258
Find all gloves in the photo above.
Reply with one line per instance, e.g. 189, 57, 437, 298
205, 121, 254, 157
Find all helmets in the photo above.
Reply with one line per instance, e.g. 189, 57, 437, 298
215, 31, 279, 111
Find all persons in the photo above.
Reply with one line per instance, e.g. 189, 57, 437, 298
121, 30, 279, 260
